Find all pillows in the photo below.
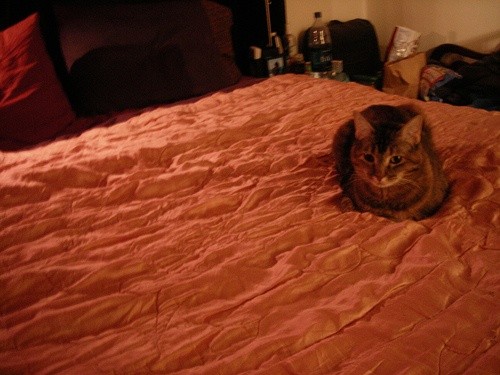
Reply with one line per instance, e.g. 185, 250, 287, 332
0, 1, 240, 141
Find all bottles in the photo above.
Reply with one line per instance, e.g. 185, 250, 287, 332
309, 12, 336, 72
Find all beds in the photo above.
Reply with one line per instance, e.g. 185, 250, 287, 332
0, 71, 500, 374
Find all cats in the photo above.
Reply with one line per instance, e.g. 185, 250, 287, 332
331, 101, 454, 220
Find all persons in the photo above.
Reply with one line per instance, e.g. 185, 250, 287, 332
272, 62, 281, 76
